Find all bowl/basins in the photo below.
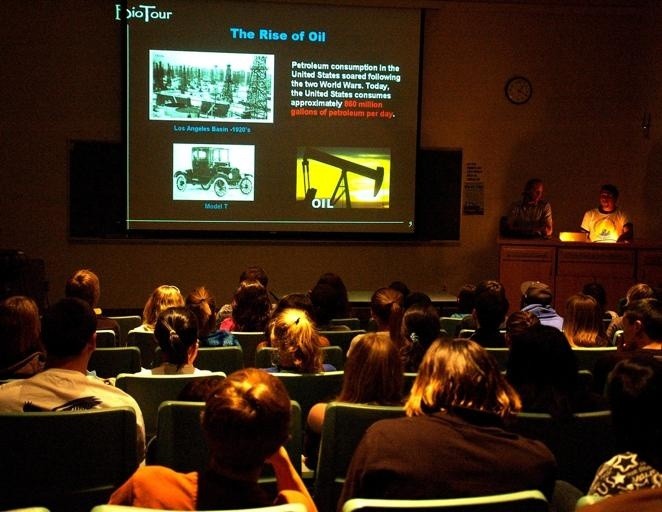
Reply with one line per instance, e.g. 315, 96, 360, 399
559, 232, 589, 244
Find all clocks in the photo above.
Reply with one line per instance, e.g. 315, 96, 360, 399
504, 74, 534, 104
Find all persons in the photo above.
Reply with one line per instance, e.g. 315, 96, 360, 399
305, 330, 406, 469
337, 334, 558, 511
1, 262, 662, 422
507, 176, 554, 241
1, 295, 149, 487
108, 366, 319, 512
586, 349, 661, 506
580, 181, 635, 244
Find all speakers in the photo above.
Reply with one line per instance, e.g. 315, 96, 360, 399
66, 138, 127, 238
414, 145, 463, 241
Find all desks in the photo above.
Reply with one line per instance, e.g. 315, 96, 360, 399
495, 239, 662, 322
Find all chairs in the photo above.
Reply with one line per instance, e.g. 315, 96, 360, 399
497, 214, 508, 238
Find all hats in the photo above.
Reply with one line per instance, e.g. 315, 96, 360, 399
521, 281, 552, 305
